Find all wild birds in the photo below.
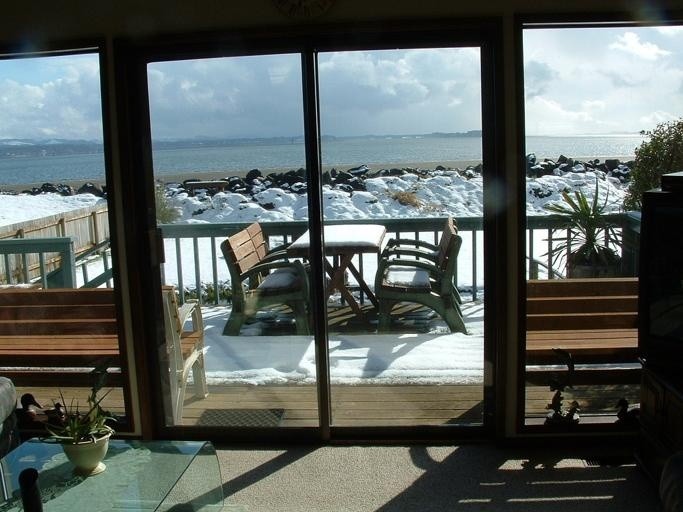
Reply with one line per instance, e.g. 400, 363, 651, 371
88, 356, 114, 374
92, 371, 108, 393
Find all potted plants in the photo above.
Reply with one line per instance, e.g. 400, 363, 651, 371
540, 173, 631, 278
43, 371, 115, 476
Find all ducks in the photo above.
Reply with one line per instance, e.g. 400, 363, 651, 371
615, 397, 641, 418
43, 402, 65, 416
15, 393, 41, 422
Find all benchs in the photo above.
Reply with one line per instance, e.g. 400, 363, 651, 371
0, 285, 209, 425
526, 279, 640, 387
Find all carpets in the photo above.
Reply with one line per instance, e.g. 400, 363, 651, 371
195, 407, 285, 427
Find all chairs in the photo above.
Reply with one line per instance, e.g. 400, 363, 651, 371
220, 216, 470, 336
